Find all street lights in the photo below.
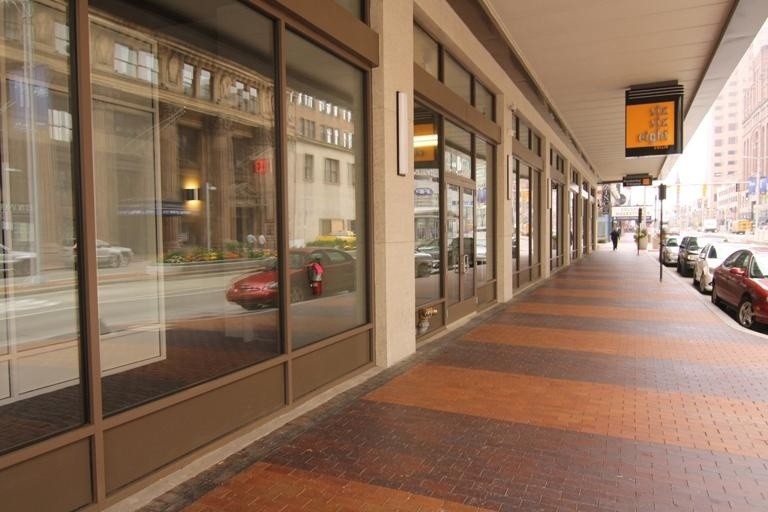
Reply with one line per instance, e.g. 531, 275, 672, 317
205, 182, 218, 249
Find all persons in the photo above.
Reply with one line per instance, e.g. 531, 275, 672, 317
610, 228, 620, 250
247, 232, 257, 250
258, 233, 265, 248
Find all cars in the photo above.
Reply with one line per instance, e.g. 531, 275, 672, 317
659, 236, 768, 329
415, 235, 516, 278
0, 244, 38, 279
225, 247, 357, 310
58, 238, 135, 270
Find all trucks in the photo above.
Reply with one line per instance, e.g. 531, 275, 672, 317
731, 219, 748, 234
703, 219, 718, 233
